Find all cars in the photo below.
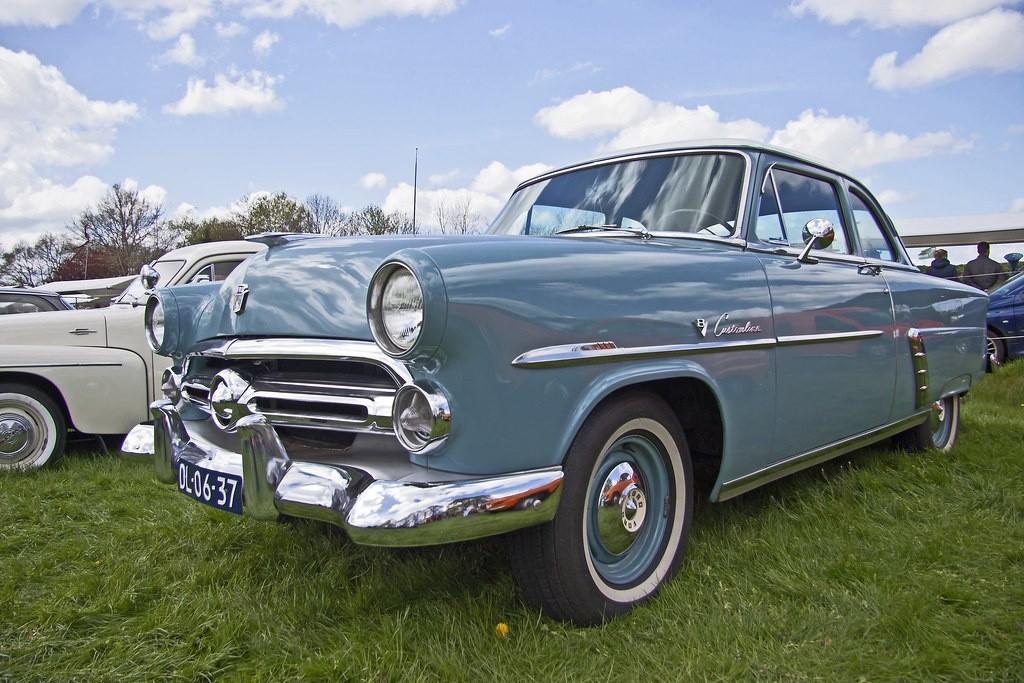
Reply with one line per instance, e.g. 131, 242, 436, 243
0, 242, 269, 473
140, 137, 989, 628
987, 270, 1024, 366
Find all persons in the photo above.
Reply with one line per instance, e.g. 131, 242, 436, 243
926, 242, 1003, 292
